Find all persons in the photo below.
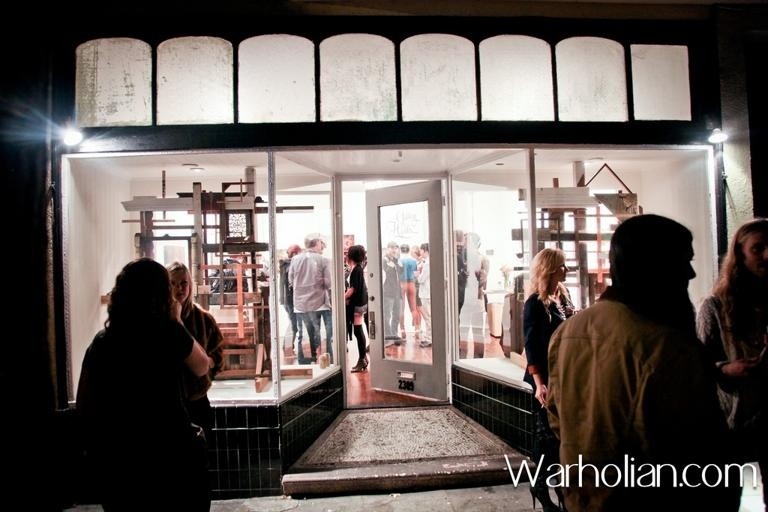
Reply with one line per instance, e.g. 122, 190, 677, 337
289, 235, 333, 366
344, 245, 369, 373
280, 245, 301, 362
382, 242, 402, 347
414, 242, 431, 348
76, 259, 214, 512
398, 245, 420, 339
455, 228, 487, 359
524, 248, 575, 510
169, 262, 223, 428
698, 219, 767, 511
546, 215, 708, 483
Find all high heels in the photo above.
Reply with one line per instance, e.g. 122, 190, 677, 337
352, 356, 368, 369
529, 478, 560, 512
351, 359, 367, 372
553, 482, 569, 512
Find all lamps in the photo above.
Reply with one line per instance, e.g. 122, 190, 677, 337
707, 127, 729, 144
